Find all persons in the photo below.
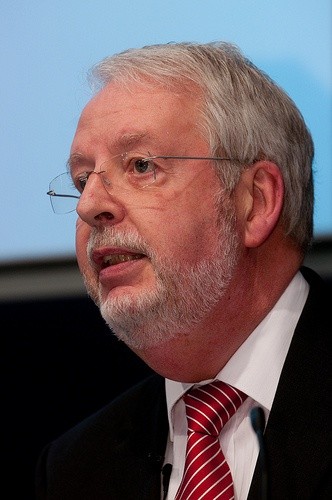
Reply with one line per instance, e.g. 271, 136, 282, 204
45, 39, 332, 498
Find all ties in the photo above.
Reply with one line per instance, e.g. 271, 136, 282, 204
174, 380, 249, 500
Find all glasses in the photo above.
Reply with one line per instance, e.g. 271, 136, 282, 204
46, 151, 260, 214
